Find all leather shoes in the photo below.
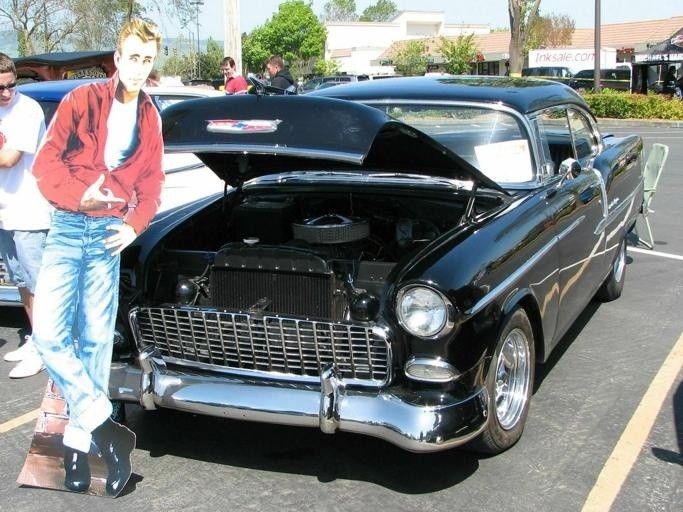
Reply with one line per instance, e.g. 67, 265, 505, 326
63, 416, 137, 498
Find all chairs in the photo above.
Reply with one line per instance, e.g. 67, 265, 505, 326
547, 139, 592, 176
625, 143, 669, 250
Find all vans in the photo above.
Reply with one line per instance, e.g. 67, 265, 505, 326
297, 74, 369, 94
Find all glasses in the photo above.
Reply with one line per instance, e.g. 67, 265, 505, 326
0, 85, 17, 94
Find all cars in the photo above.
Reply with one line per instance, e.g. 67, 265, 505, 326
109, 72, 645, 454
521, 66, 572, 84
567, 69, 630, 91
0, 77, 225, 310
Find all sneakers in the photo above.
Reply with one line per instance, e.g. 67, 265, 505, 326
3, 334, 46, 378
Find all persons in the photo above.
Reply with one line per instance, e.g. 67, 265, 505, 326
265, 54, 296, 92
221, 56, 248, 95
662, 66, 676, 97
0, 51, 50, 380
30, 16, 163, 499
